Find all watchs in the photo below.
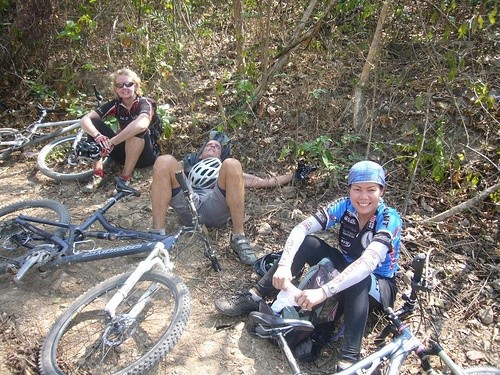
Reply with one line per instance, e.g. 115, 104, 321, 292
325, 282, 338, 296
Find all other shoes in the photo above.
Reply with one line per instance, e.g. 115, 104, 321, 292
115, 176, 130, 192
82, 174, 104, 193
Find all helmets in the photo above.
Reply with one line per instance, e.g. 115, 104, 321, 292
187, 157, 223, 190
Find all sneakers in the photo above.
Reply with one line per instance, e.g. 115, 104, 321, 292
142, 228, 166, 244
229, 230, 261, 265
335, 358, 357, 375
214, 290, 266, 317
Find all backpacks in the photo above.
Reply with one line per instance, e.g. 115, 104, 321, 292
259, 255, 351, 363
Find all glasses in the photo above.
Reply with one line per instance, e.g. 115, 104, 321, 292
114, 81, 137, 90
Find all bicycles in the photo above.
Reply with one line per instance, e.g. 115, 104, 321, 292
0, 84, 110, 181
1, 170, 221, 375
245, 254, 500, 375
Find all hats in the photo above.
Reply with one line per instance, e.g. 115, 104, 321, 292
347, 161, 388, 186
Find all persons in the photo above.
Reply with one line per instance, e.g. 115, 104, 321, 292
215, 161, 405, 375
82, 70, 160, 196
134, 139, 304, 266
